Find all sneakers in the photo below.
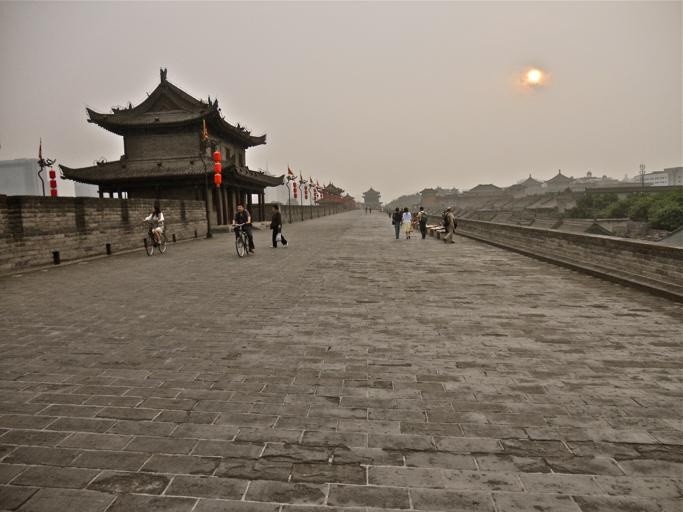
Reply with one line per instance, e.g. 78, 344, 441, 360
158, 240, 162, 243
237, 243, 242, 247
250, 249, 256, 253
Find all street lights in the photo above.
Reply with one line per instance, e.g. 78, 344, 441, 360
36, 159, 50, 197
284, 175, 292, 224
307, 184, 343, 219
196, 139, 218, 239
298, 181, 306, 222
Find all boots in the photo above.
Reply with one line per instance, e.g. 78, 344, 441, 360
406, 234, 408, 239
408, 233, 410, 239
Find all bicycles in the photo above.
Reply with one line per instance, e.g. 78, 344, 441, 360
142, 219, 168, 257
231, 221, 252, 257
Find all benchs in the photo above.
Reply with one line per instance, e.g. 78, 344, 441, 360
413, 223, 446, 240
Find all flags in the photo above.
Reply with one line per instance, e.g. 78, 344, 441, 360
287, 165, 325, 189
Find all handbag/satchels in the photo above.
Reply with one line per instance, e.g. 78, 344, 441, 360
392, 220, 395, 225
410, 226, 413, 232
276, 232, 282, 242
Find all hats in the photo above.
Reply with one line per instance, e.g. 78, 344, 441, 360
447, 207, 451, 209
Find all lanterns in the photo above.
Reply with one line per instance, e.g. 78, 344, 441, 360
213, 174, 222, 188
50, 180, 56, 188
213, 164, 221, 173
293, 182, 345, 207
50, 189, 57, 198
48, 171, 55, 178
212, 151, 221, 163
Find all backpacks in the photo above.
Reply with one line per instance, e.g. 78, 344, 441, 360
443, 211, 450, 226
420, 211, 428, 222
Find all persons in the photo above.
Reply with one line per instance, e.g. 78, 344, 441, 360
365, 205, 457, 244
269, 205, 288, 247
233, 205, 255, 253
143, 206, 164, 245
